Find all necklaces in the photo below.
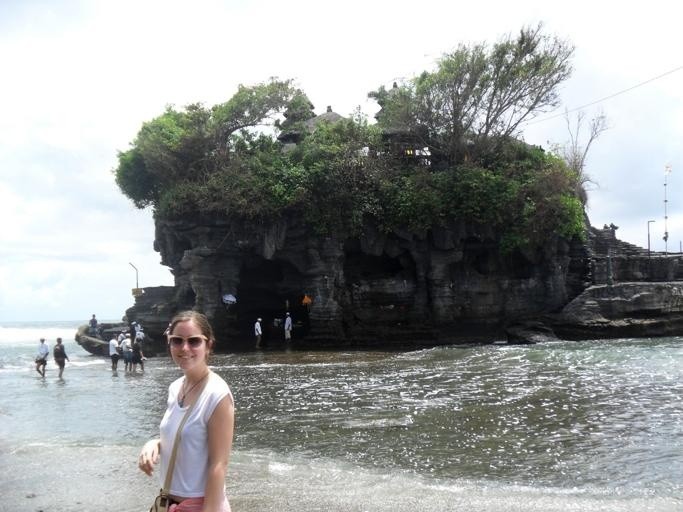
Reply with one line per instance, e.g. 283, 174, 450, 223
178, 371, 210, 408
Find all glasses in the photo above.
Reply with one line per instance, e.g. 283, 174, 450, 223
167, 335, 209, 348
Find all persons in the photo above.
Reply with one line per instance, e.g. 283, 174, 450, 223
254, 317, 262, 348
54, 338, 70, 377
139, 311, 234, 512
109, 320, 147, 377
90, 314, 97, 338
273, 311, 293, 340
35, 338, 49, 377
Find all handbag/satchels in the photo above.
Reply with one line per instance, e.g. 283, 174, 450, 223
150, 496, 169, 512
